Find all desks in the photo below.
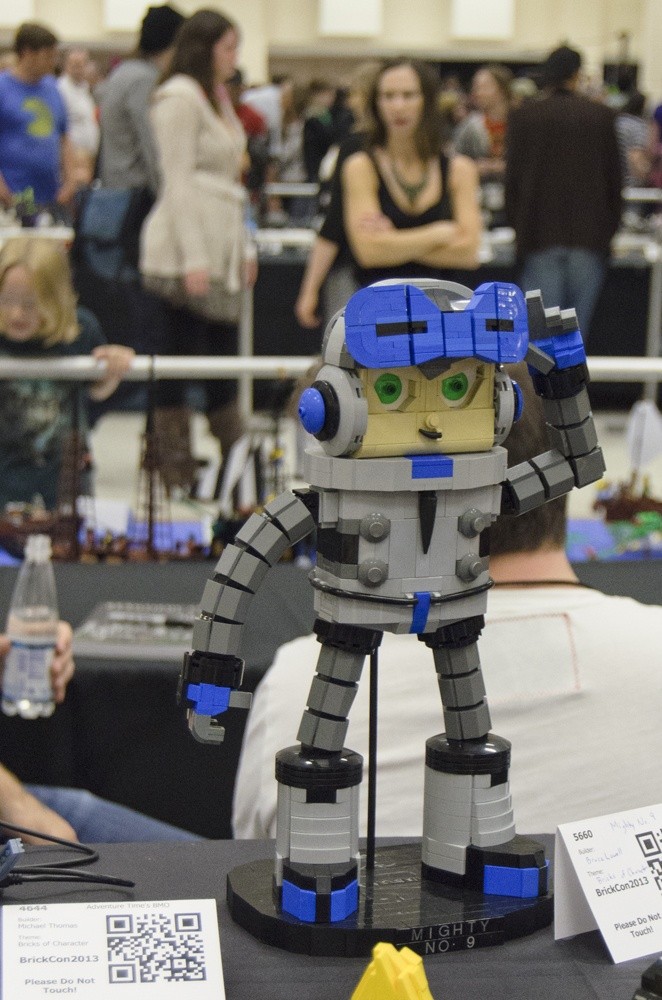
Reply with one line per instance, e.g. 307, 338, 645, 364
250, 230, 662, 408
0, 555, 314, 841
0, 829, 662, 1000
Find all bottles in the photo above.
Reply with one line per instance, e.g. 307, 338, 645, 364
0, 532, 57, 719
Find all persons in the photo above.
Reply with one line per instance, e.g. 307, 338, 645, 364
73, 6, 662, 413
502, 45, 623, 347
0, 621, 212, 848
0, 44, 101, 291
232, 362, 662, 839
137, 10, 260, 487
0, 22, 69, 230
0, 233, 134, 561
293, 88, 361, 341
341, 58, 483, 289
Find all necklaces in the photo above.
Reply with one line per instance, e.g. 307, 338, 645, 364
486, 580, 593, 591
388, 151, 431, 207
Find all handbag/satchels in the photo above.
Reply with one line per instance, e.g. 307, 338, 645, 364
116, 184, 158, 263
